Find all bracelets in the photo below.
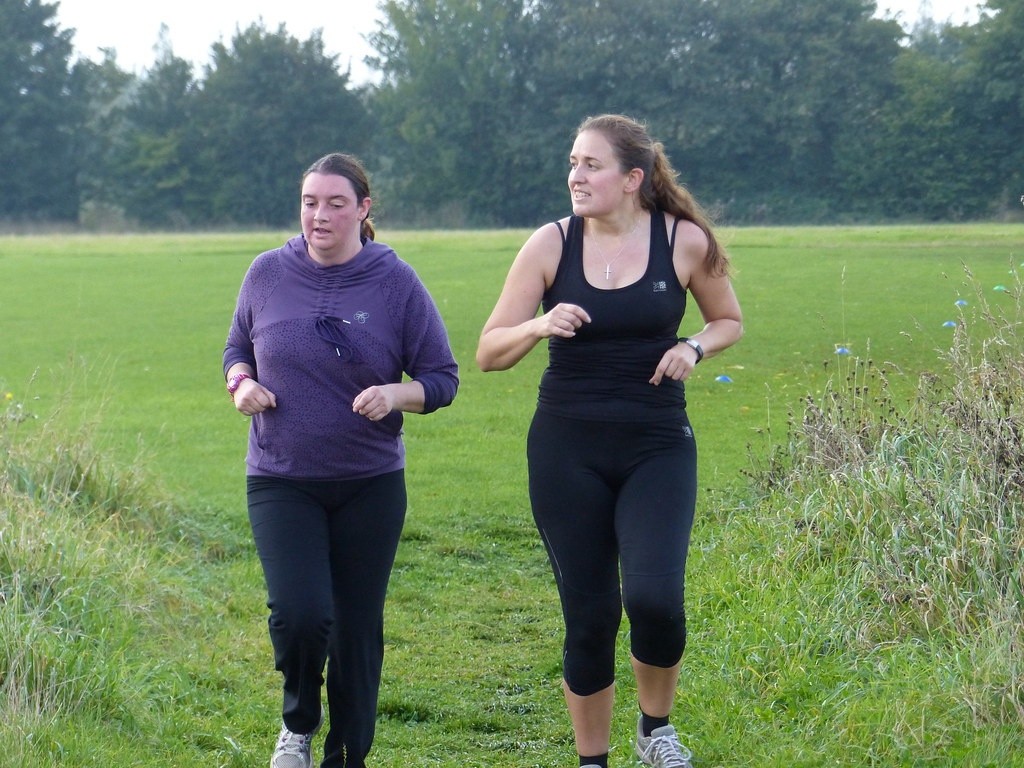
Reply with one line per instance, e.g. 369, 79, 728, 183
678, 337, 704, 365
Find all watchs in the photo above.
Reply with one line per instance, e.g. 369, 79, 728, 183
227, 373, 253, 403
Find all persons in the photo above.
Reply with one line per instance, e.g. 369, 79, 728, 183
221, 153, 461, 768
475, 113, 745, 768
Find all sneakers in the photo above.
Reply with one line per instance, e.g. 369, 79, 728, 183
269, 701, 326, 768
634, 714, 694, 768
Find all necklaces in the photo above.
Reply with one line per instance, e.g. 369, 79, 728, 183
587, 209, 641, 281
